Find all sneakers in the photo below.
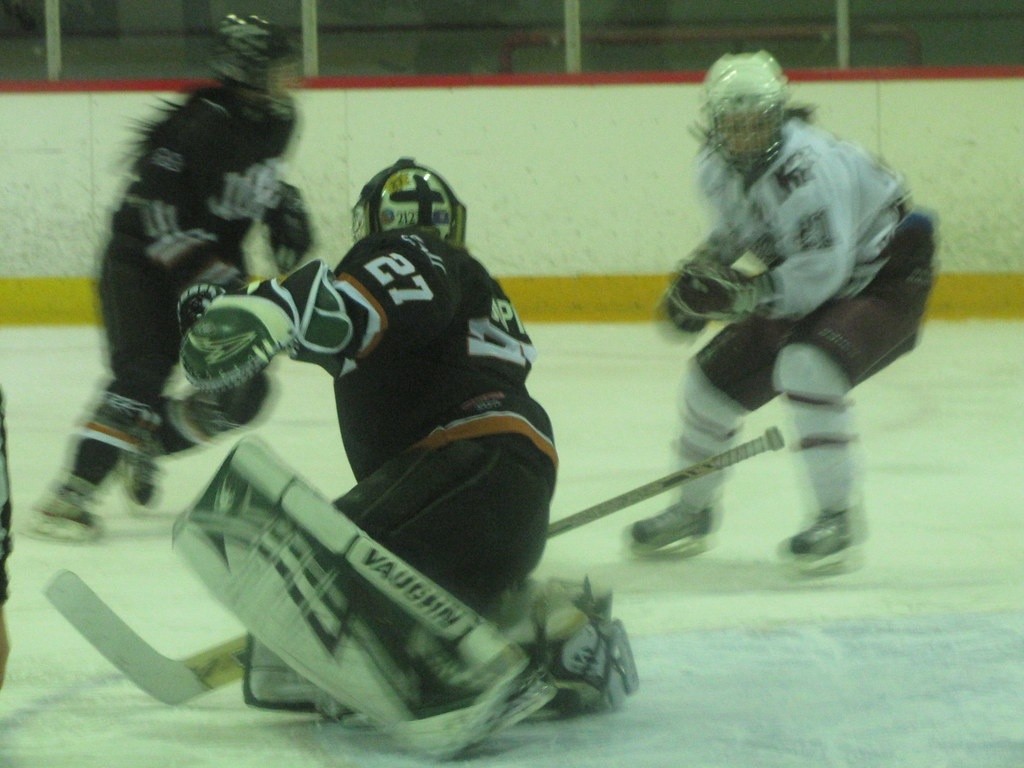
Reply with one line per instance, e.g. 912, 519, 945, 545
792, 509, 853, 574
631, 501, 714, 561
29, 476, 103, 543
115, 408, 166, 511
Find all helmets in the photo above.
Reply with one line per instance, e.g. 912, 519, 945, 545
352, 159, 466, 245
211, 15, 289, 94
702, 49, 788, 173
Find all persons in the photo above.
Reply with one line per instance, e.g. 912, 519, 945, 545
31, 13, 314, 544
172, 157, 640, 759
0, 389, 13, 687
630, 50, 940, 577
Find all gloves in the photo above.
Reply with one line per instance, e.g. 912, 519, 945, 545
146, 230, 241, 295
664, 251, 775, 332
262, 182, 314, 274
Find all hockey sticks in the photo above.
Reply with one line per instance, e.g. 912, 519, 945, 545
44, 425, 785, 707
